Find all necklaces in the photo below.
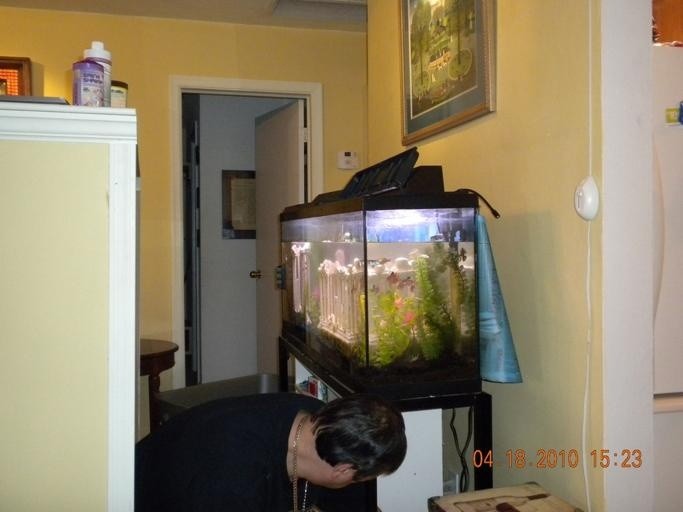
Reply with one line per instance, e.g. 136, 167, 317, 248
293, 416, 308, 510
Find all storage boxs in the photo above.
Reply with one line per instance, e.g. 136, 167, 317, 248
427, 481, 585, 512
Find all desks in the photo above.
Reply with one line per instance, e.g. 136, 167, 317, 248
140, 339, 179, 433
277, 334, 493, 511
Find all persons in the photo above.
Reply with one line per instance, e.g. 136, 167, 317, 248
134, 392, 406, 511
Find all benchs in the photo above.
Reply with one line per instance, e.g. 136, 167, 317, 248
150, 375, 256, 430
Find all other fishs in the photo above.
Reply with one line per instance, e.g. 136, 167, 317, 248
395, 297, 405, 309
351, 271, 416, 295
405, 352, 420, 362
403, 311, 416, 324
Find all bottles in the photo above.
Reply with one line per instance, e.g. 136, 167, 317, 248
71, 41, 129, 106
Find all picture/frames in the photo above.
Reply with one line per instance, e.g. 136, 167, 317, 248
396, 0, 497, 147
221, 170, 257, 240
0, 56, 32, 96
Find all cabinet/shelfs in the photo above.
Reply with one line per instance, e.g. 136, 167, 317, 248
0, 102, 139, 512
